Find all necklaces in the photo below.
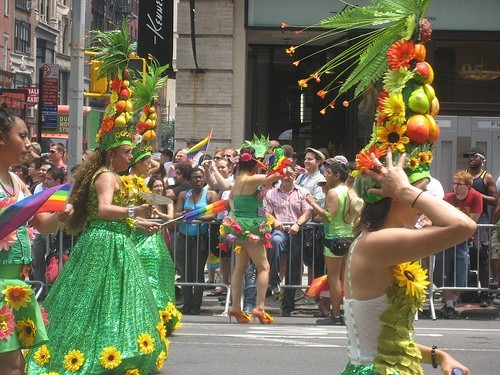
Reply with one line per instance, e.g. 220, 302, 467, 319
0, 174, 16, 196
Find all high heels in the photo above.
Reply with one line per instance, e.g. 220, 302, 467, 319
251, 307, 274, 323
229, 309, 251, 324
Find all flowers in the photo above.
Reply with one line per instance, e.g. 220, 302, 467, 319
0, 0, 444, 375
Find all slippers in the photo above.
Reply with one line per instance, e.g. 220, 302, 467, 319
207, 290, 227, 296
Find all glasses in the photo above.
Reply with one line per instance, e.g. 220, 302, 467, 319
49, 150, 60, 155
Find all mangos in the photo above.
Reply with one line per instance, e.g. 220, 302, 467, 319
406, 43, 440, 144
116, 80, 133, 112
143, 106, 157, 140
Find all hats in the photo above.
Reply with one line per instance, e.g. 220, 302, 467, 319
158, 149, 173, 158
305, 147, 325, 163
334, 155, 348, 165
323, 158, 336, 166
343, 189, 365, 223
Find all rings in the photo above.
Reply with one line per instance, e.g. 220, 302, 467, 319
377, 164, 384, 171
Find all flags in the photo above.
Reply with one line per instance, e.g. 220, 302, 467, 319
0, 182, 73, 240
184, 200, 226, 222
186, 128, 212, 159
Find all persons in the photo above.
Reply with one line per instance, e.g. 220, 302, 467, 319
128, 141, 182, 336
11, 142, 95, 301
23, 130, 169, 375
149, 136, 359, 325
0, 106, 74, 375
418, 149, 500, 318
342, 147, 476, 375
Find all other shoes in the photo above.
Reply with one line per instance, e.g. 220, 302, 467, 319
318, 303, 332, 317
316, 315, 342, 325
479, 291, 490, 307
192, 308, 202, 315
492, 290, 500, 304
266, 289, 272, 296
274, 292, 280, 300
179, 307, 191, 315
281, 308, 292, 316
438, 305, 455, 319
279, 287, 284, 301
203, 282, 216, 290
294, 290, 304, 301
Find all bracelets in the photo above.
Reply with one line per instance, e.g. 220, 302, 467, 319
296, 222, 301, 227
128, 205, 135, 218
411, 189, 426, 208
431, 345, 437, 368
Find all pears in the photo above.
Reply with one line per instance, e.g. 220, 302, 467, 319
115, 111, 131, 127
408, 84, 429, 114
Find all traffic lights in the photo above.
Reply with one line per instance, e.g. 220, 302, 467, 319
126, 58, 148, 95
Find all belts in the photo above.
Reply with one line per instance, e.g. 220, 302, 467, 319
180, 233, 207, 240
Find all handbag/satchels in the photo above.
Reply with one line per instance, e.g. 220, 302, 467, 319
327, 238, 350, 256
45, 253, 68, 283
